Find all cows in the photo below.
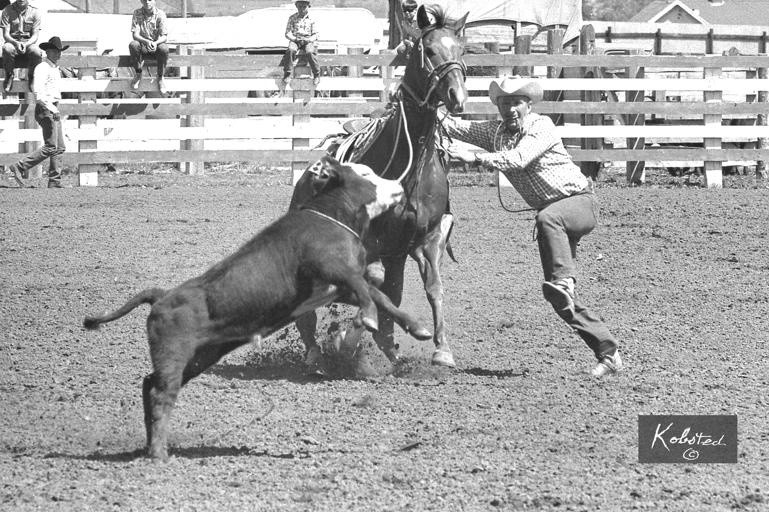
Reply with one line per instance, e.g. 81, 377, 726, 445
82, 154, 434, 459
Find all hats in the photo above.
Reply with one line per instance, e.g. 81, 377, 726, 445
39, 36, 71, 51
487, 74, 545, 105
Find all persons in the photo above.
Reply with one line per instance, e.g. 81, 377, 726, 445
394, 1, 420, 55
282, 2, 321, 86
434, 76, 622, 380
127, 0, 169, 99
0, 0, 44, 95
9, 37, 71, 190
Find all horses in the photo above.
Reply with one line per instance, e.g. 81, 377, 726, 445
288, 2, 471, 369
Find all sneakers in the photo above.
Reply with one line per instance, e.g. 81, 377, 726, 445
131, 71, 168, 97
3, 72, 15, 93
282, 72, 320, 86
10, 164, 26, 187
587, 348, 622, 382
46, 181, 75, 188
542, 279, 576, 326
